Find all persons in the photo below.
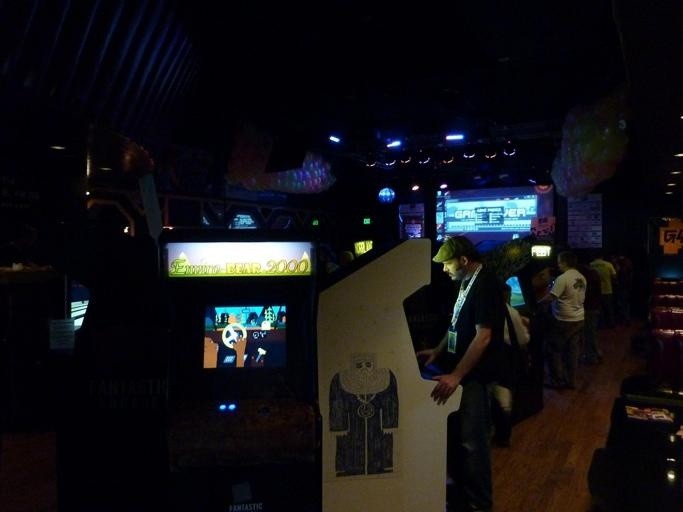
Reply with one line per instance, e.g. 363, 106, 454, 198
589, 252, 617, 330
503, 303, 531, 348
611, 254, 635, 327
416, 235, 507, 512
537, 250, 588, 389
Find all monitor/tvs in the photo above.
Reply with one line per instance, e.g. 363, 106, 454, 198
505, 273, 526, 308
203, 302, 291, 382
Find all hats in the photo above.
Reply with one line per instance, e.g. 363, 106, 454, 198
433, 236, 471, 263
